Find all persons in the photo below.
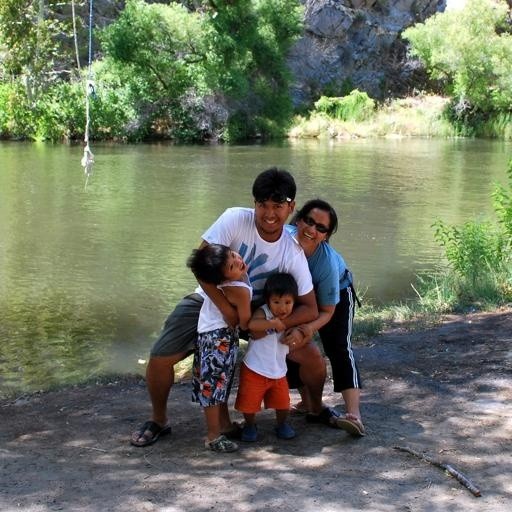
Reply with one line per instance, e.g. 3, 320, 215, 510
234, 273, 297, 442
187, 242, 253, 453
129, 168, 345, 447
281, 199, 366, 438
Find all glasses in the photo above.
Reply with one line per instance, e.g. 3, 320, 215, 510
256, 193, 292, 204
303, 216, 329, 234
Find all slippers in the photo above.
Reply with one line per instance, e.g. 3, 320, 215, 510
305, 406, 329, 424
205, 435, 238, 453
273, 423, 295, 439
290, 405, 305, 417
221, 422, 241, 440
241, 423, 258, 441
337, 419, 366, 437
129, 421, 172, 447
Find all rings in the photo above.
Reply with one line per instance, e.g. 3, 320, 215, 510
293, 341, 297, 346
